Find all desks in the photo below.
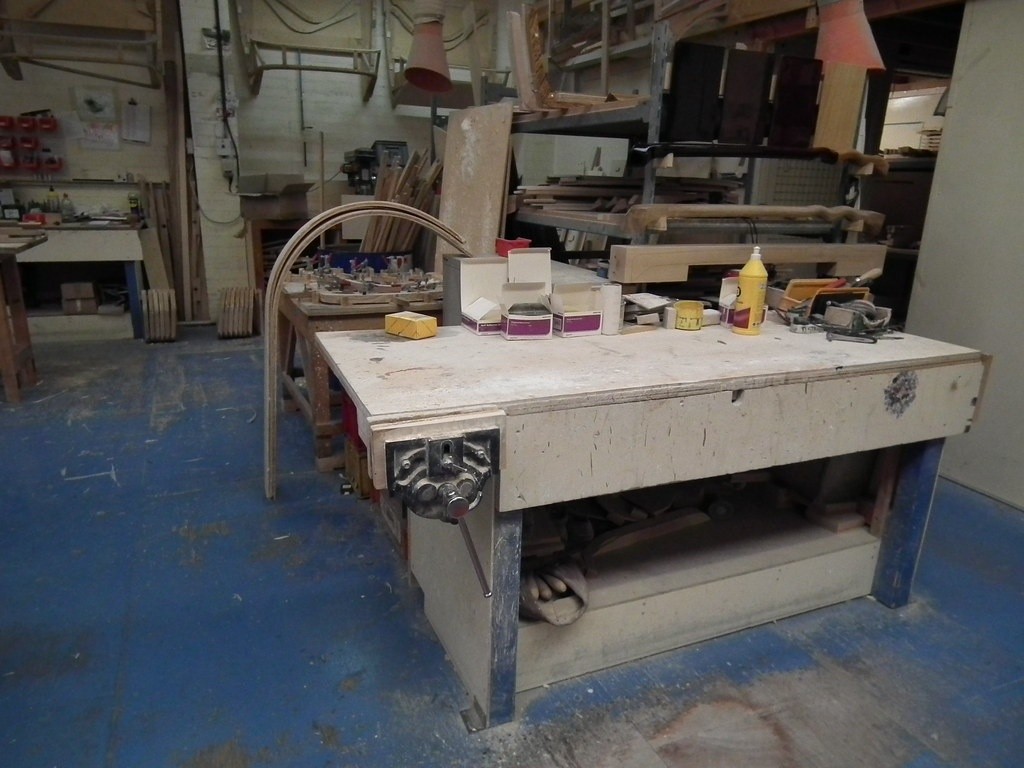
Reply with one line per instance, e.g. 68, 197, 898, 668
316, 310, 995, 727
278, 280, 443, 473
0, 229, 48, 402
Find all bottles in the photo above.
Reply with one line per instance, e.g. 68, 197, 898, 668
60, 193, 74, 223
47, 184, 59, 211
732, 245, 768, 336
